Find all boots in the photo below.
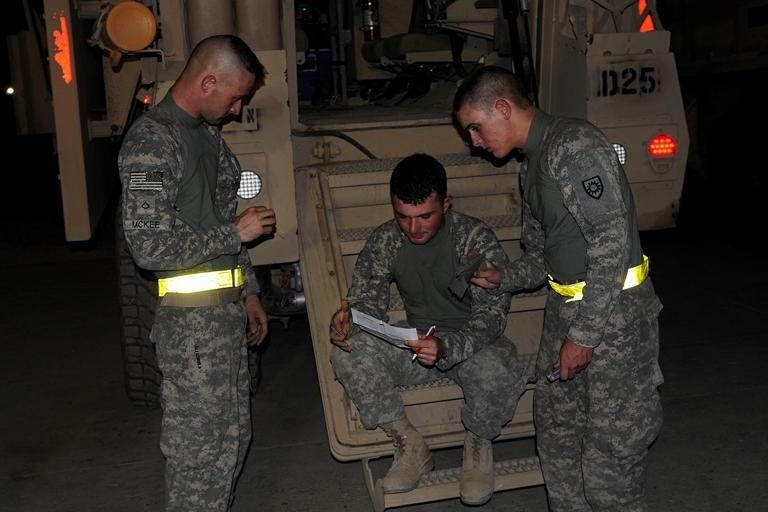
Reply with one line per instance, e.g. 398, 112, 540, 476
378, 418, 434, 494
458, 430, 494, 505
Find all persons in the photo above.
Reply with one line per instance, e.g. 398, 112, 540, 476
454, 65, 664, 512
330, 152, 528, 506
118, 36, 276, 512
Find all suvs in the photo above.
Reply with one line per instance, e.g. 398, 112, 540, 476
44, 1, 690, 512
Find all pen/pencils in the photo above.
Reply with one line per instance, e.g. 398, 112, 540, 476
411, 325, 437, 361
